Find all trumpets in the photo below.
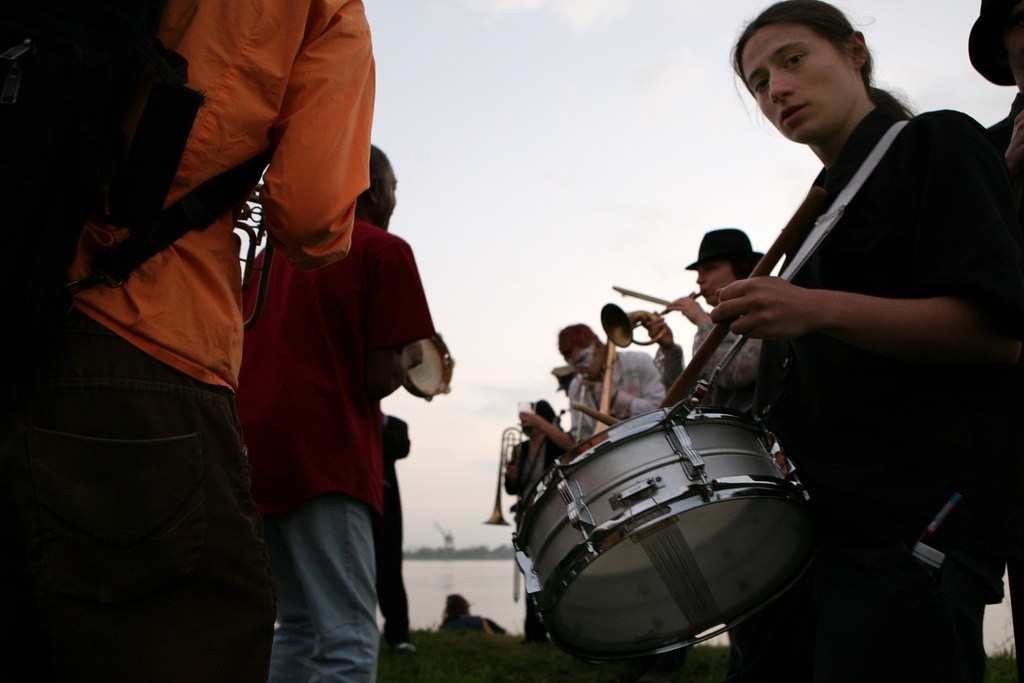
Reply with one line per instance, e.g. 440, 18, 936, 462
601, 287, 703, 348
484, 427, 523, 526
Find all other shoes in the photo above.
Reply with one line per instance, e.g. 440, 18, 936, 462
390, 642, 417, 652
521, 635, 548, 644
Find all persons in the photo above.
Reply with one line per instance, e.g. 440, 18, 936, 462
0, 0, 375, 683
437, 594, 512, 634
503, 0, 1024, 683
235, 144, 435, 682
374, 412, 416, 655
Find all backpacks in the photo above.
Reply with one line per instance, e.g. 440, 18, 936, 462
0, 0, 274, 314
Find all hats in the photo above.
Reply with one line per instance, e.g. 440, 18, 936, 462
686, 228, 765, 270
551, 363, 576, 391
969, 0, 1024, 86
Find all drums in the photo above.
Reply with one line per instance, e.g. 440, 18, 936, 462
514, 402, 822, 662
